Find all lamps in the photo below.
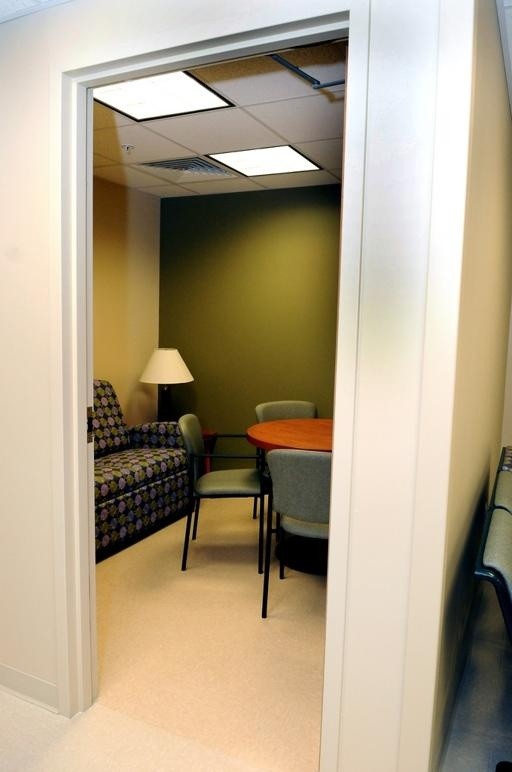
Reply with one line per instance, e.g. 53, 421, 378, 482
139, 348, 195, 422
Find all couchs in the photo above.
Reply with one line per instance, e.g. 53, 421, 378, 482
93, 380, 205, 563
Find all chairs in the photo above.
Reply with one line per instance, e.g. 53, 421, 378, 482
473, 508, 512, 660
261, 449, 330, 619
178, 412, 265, 573
490, 470, 512, 516
252, 399, 318, 519
498, 445, 512, 471
